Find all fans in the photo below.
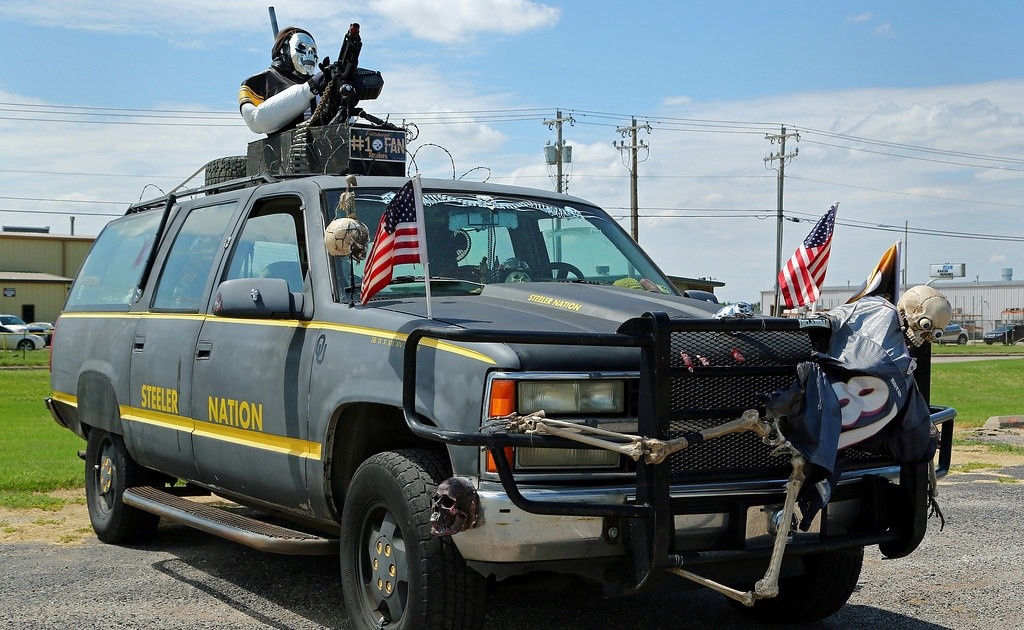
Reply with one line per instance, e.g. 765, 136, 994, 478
427, 228, 472, 277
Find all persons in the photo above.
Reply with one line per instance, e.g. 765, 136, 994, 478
239, 27, 356, 138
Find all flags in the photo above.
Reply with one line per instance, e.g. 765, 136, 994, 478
777, 205, 836, 310
359, 176, 426, 306
845, 240, 900, 307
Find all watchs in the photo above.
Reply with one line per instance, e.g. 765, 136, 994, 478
307, 78, 320, 95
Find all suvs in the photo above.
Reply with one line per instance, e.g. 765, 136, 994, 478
44, 172, 957, 630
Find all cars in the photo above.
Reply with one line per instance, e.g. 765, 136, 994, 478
932, 322, 969, 345
983, 323, 1015, 345
0, 314, 55, 351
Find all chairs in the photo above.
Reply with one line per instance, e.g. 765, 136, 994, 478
256, 261, 364, 293
